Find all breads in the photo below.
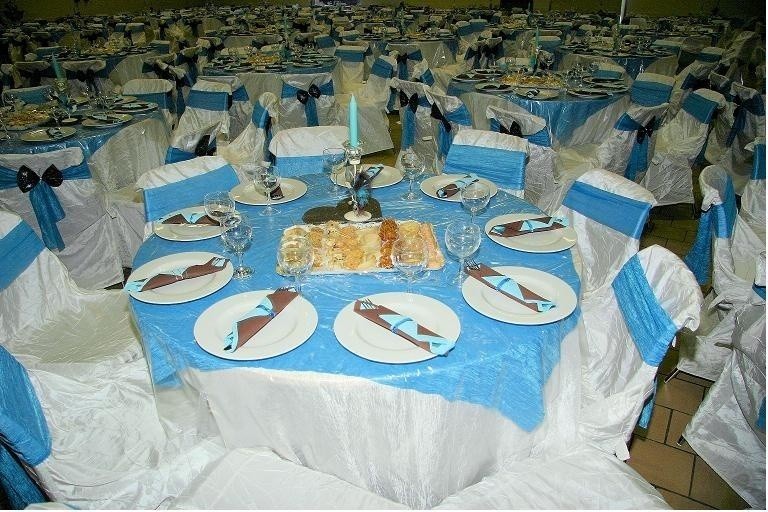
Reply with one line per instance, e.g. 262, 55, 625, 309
280, 221, 443, 271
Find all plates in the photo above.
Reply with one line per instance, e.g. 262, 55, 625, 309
124, 249, 235, 307
192, 288, 320, 359
0, 3, 732, 148
126, 149, 582, 297
458, 264, 579, 326
333, 290, 462, 365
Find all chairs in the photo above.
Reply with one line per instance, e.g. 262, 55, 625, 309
662, 133, 767, 510
0, 126, 704, 510
0, 78, 174, 184
390, 57, 722, 226
0, 75, 234, 291
154, 43, 398, 157
